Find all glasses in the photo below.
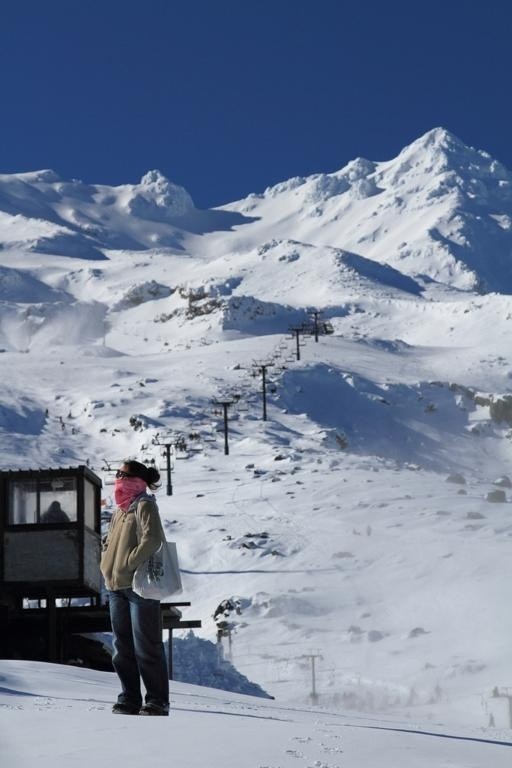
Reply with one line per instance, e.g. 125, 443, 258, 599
116, 469, 135, 482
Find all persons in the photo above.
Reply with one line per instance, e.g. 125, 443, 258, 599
39, 499, 71, 524
98, 460, 171, 717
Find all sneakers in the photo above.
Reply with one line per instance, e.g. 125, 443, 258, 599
112, 702, 143, 715
139, 700, 171, 716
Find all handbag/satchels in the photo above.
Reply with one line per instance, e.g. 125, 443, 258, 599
131, 500, 183, 600
